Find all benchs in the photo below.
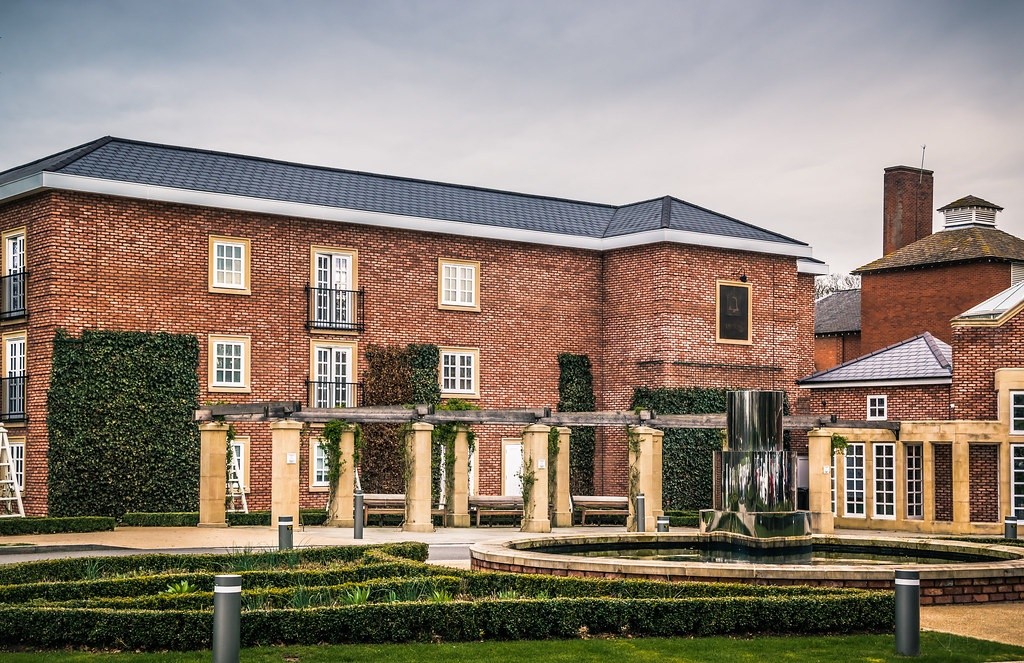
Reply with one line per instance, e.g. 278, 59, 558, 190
570, 495, 629, 526
469, 495, 553, 528
353, 493, 449, 528
